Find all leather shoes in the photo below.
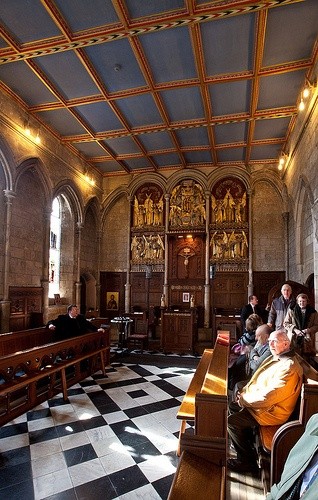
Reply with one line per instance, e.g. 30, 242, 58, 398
227, 459, 259, 472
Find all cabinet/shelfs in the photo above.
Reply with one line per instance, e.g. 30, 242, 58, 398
160, 307, 242, 355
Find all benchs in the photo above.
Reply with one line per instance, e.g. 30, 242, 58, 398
262, 351, 318, 495
0, 325, 109, 400
176, 349, 214, 457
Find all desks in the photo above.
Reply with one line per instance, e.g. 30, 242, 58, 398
111, 320, 133, 350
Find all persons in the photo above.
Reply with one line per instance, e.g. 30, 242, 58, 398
283, 294, 318, 370
267, 284, 295, 332
228, 314, 263, 391
228, 330, 303, 471
232, 324, 273, 401
241, 295, 262, 335
46, 305, 105, 343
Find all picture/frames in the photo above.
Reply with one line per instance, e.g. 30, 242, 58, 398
182, 293, 190, 302
106, 291, 120, 311
